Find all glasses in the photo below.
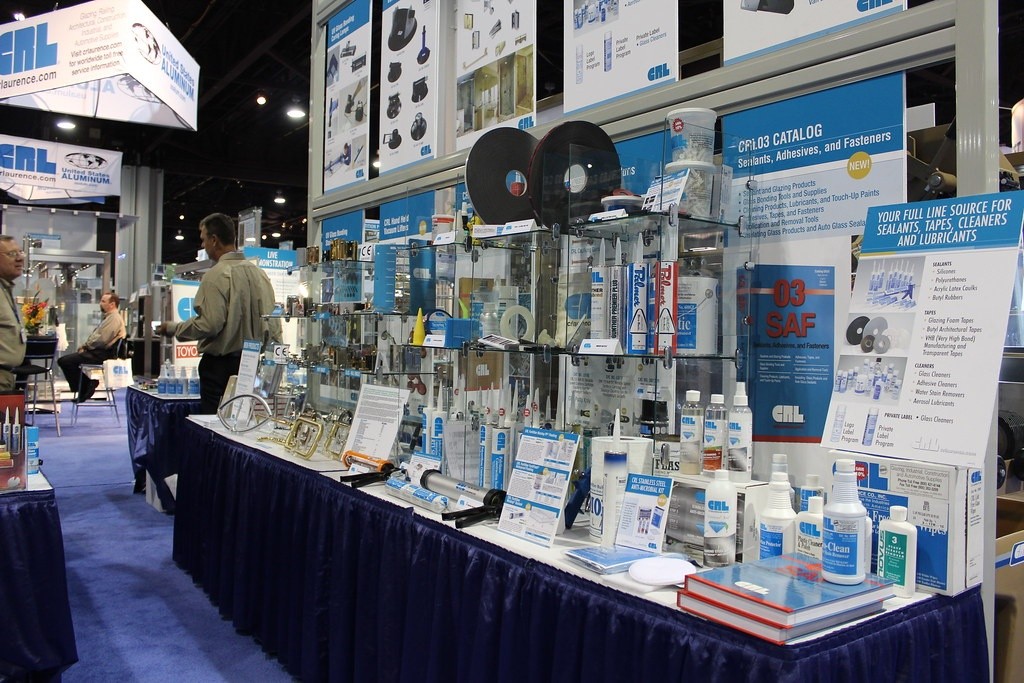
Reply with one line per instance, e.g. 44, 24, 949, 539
0, 249, 24, 256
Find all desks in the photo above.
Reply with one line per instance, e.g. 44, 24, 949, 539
0, 467, 78, 683
125, 385, 201, 516
21, 332, 59, 414
173, 416, 989, 683
127, 337, 160, 375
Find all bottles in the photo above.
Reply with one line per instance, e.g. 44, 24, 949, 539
834, 358, 902, 401
728, 382, 752, 482
703, 394, 728, 470
26, 427, 39, 474
703, 469, 737, 569
574, 0, 620, 83
866, 260, 919, 310
759, 453, 918, 598
679, 390, 704, 476
0, 407, 21, 455
423, 378, 628, 547
830, 404, 846, 442
158, 359, 200, 396
471, 233, 676, 357
862, 407, 878, 446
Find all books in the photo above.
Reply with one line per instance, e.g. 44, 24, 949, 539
677, 551, 897, 646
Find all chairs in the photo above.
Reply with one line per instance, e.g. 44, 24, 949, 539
11, 338, 61, 437
70, 338, 122, 429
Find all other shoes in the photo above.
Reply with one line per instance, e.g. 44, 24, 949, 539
72, 391, 86, 404
85, 379, 99, 400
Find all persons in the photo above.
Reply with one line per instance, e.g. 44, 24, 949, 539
153, 214, 284, 413
57, 292, 127, 403
0, 234, 27, 445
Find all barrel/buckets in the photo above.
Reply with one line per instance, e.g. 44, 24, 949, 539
665, 161, 717, 217
677, 275, 721, 354
667, 108, 718, 163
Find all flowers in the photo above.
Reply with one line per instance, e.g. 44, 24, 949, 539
20, 289, 49, 335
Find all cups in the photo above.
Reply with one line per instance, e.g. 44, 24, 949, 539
271, 238, 375, 369
432, 214, 454, 245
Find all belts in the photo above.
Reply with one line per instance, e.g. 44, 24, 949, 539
0, 364, 16, 373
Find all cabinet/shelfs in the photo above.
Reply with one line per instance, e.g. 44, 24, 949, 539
262, 117, 753, 513
